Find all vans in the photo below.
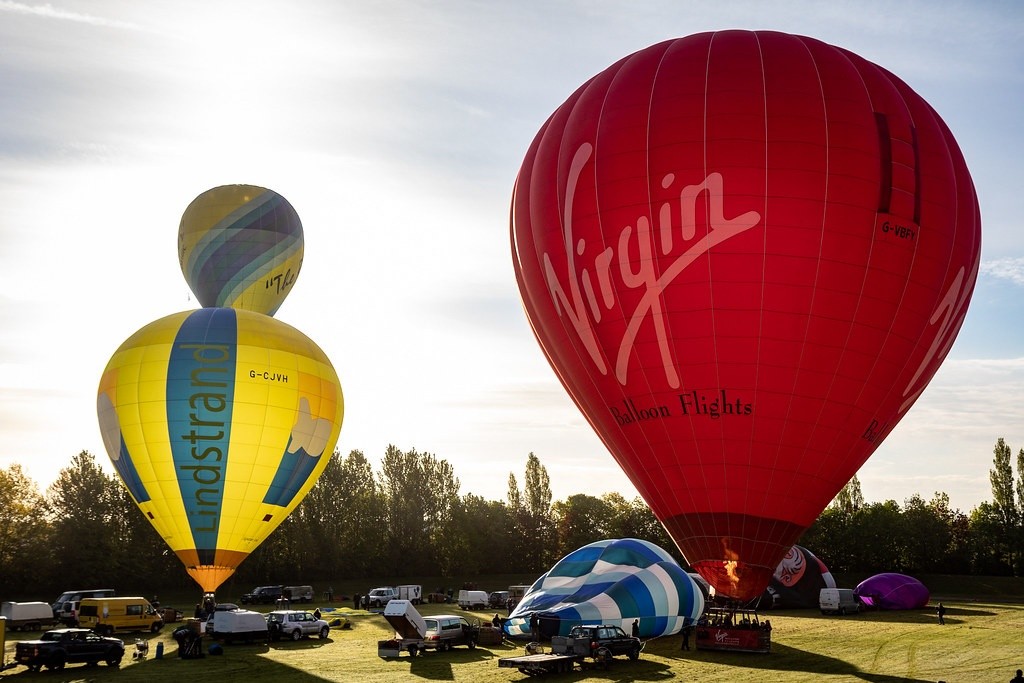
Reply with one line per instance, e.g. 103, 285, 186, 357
77, 597, 166, 636
508, 585, 532, 606
820, 588, 862, 616
51, 589, 116, 624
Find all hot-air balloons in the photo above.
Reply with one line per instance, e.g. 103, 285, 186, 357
96, 306, 345, 613
503, 537, 713, 644
506, 25, 983, 653
176, 183, 306, 319
851, 573, 931, 614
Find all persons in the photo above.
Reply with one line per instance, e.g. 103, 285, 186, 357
206, 600, 215, 613
447, 588, 454, 598
282, 586, 290, 609
632, 619, 640, 637
508, 599, 516, 615
328, 586, 333, 602
529, 613, 539, 642
697, 615, 770, 631
353, 592, 361, 610
935, 603, 945, 625
313, 609, 321, 621
195, 604, 205, 620
1010, 669, 1024, 683
681, 618, 691, 651
364, 595, 370, 611
173, 625, 192, 657
150, 596, 160, 609
492, 613, 501, 627
709, 594, 713, 600
759, 598, 771, 612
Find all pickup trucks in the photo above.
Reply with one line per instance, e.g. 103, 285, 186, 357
14, 628, 127, 671
361, 587, 399, 608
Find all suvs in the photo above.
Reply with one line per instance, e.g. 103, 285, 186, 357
241, 586, 284, 605
282, 585, 314, 604
265, 609, 330, 642
59, 600, 82, 627
421, 614, 481, 653
487, 591, 509, 609
568, 623, 642, 664
